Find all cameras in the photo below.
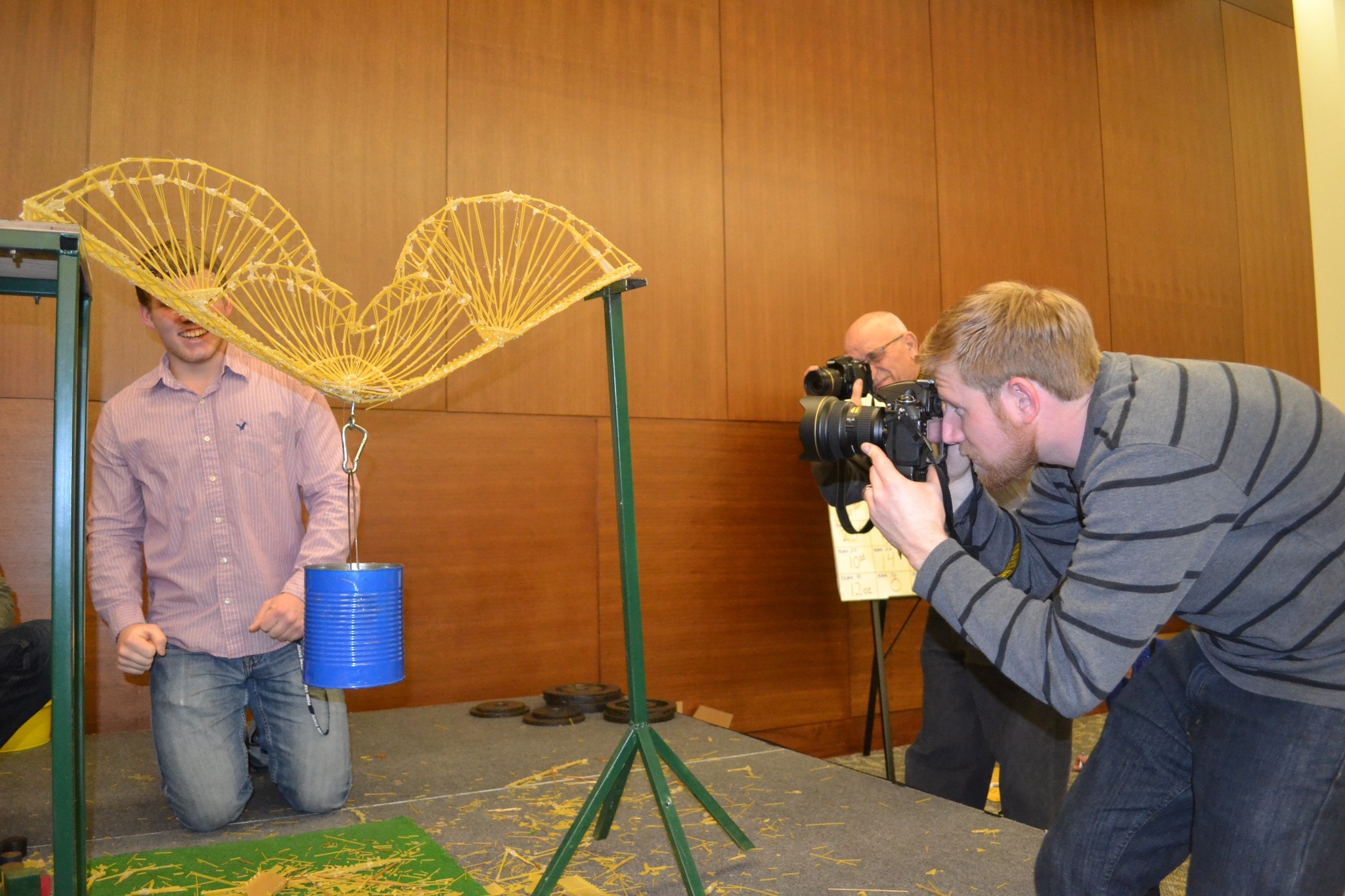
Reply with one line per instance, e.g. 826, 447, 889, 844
803, 354, 871, 401
798, 380, 946, 483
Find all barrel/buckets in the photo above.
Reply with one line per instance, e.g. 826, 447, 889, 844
302, 468, 406, 689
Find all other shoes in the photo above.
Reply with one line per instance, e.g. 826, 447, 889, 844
245, 718, 269, 770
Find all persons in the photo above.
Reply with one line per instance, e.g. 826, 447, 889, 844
88, 240, 361, 831
860, 281, 1345, 895
804, 312, 1071, 830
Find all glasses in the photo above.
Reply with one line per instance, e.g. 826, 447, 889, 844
863, 334, 905, 365
